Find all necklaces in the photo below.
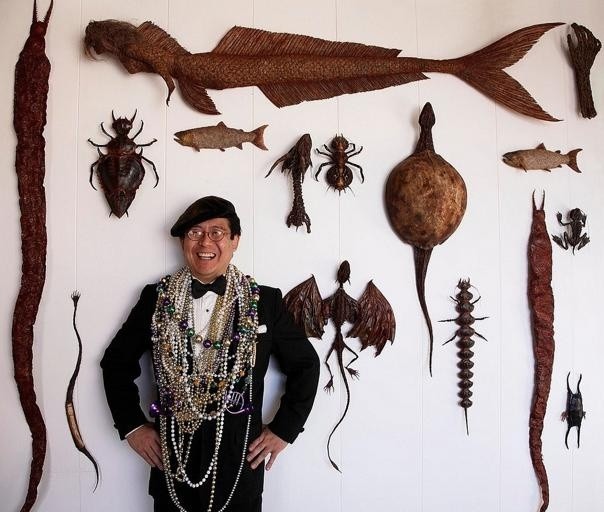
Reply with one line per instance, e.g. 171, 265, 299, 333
146, 261, 263, 511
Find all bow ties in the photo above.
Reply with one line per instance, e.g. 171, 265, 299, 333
191, 275, 227, 298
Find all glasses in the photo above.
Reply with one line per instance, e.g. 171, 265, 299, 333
186, 229, 232, 242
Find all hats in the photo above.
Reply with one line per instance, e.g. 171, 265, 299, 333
171, 196, 235, 237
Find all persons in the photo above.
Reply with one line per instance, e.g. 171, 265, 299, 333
566, 21, 602, 121
96, 193, 319, 512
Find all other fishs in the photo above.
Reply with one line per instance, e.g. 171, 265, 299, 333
174, 121, 269, 152
503, 143, 582, 173
